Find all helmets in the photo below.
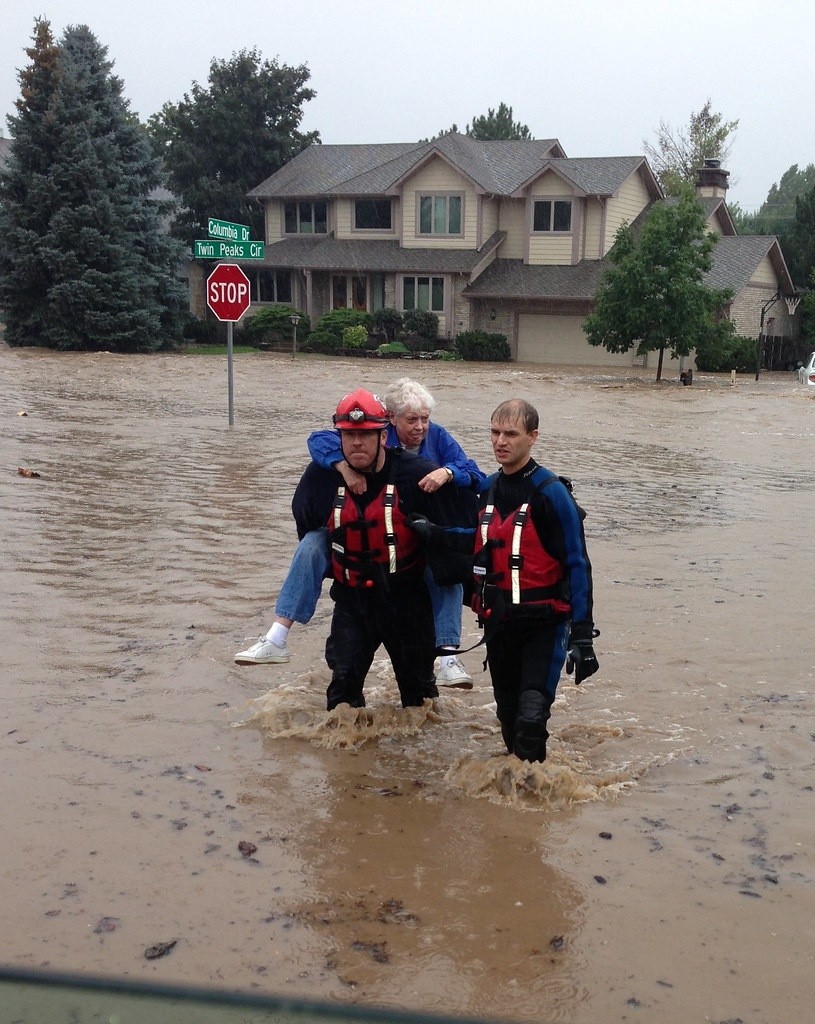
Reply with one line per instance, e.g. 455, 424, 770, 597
334, 389, 389, 429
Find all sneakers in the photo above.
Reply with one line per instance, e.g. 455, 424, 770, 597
234, 635, 290, 665
435, 659, 473, 689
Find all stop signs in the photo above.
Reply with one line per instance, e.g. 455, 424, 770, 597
205, 264, 251, 323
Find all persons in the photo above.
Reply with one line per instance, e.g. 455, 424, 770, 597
291, 389, 481, 711
474, 401, 600, 762
234, 376, 486, 693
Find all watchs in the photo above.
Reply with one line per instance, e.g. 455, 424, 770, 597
442, 466, 453, 482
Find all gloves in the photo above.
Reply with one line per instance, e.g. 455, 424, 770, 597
404, 511, 444, 542
565, 620, 600, 684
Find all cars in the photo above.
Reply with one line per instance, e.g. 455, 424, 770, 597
796, 352, 815, 386
